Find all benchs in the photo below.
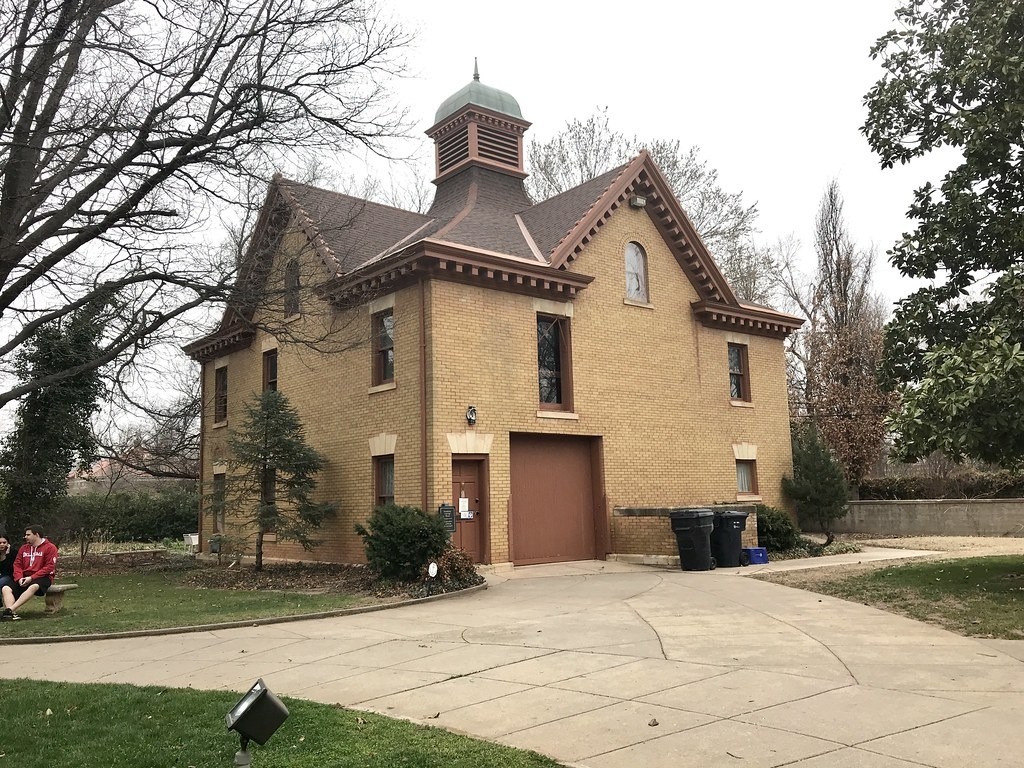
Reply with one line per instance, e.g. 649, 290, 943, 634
44, 584, 79, 616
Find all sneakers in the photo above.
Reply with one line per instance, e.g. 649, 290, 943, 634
3, 609, 20, 621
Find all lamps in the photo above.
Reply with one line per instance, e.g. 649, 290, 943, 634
466, 405, 478, 427
630, 195, 646, 208
224, 677, 291, 765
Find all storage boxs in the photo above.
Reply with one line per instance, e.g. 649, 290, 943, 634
742, 546, 770, 565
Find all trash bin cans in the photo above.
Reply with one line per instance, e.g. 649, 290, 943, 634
670, 508, 718, 571
711, 511, 750, 568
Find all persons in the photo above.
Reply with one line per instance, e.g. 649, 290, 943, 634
1, 523, 59, 620
0, 532, 19, 609
